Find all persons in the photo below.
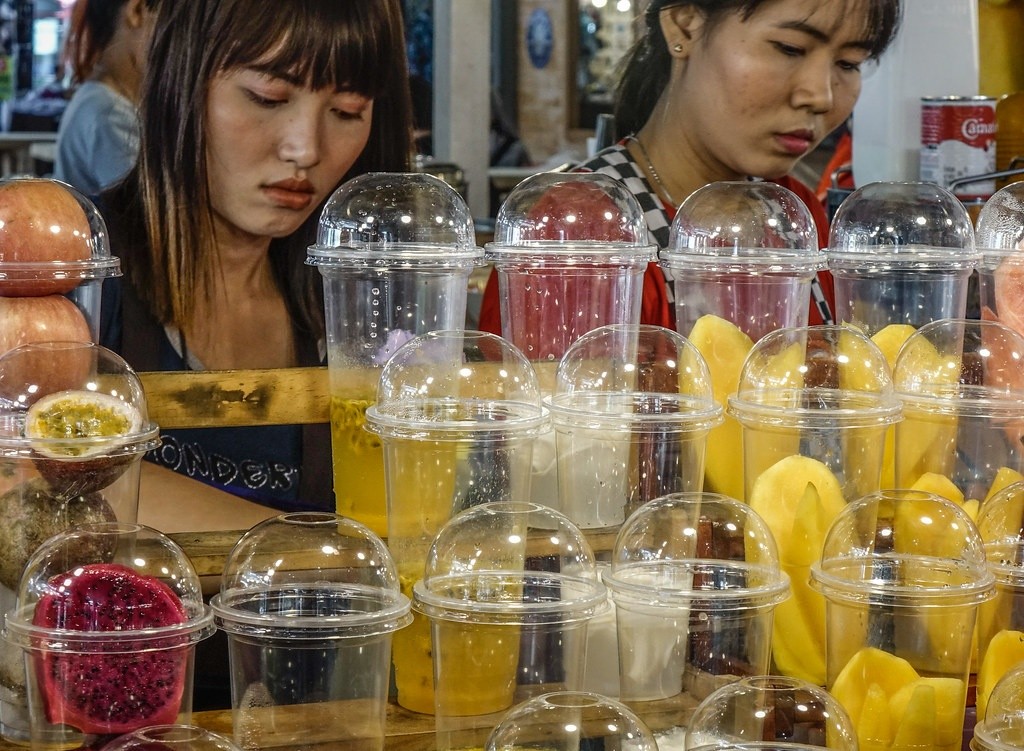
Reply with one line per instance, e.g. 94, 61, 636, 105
71, 0, 550, 712
51, 0, 162, 196
479, 0, 902, 398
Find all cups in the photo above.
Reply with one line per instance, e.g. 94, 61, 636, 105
0, 172, 1024, 751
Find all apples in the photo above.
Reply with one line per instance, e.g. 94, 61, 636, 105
0, 295, 93, 409
0, 178, 92, 297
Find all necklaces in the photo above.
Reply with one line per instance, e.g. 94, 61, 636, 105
636, 137, 678, 210
